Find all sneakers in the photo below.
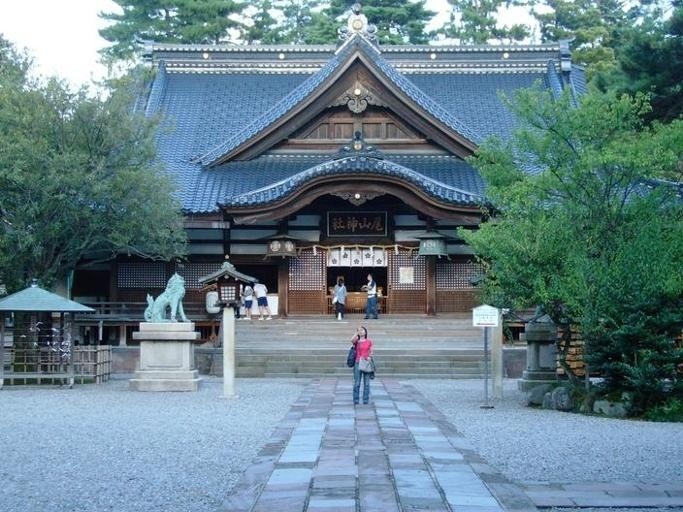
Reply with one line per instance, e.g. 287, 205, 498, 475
363, 315, 378, 320
257, 316, 273, 321
354, 399, 370, 406
235, 316, 251, 322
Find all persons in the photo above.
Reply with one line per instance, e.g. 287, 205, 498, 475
241, 282, 254, 320
362, 273, 379, 320
253, 282, 272, 320
235, 284, 244, 319
329, 278, 346, 320
350, 325, 374, 404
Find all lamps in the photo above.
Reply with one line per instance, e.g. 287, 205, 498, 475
353, 131, 362, 151
414, 228, 453, 261
260, 228, 302, 262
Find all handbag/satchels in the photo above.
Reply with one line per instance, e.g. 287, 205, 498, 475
332, 295, 338, 305
346, 345, 357, 368
358, 356, 376, 378
